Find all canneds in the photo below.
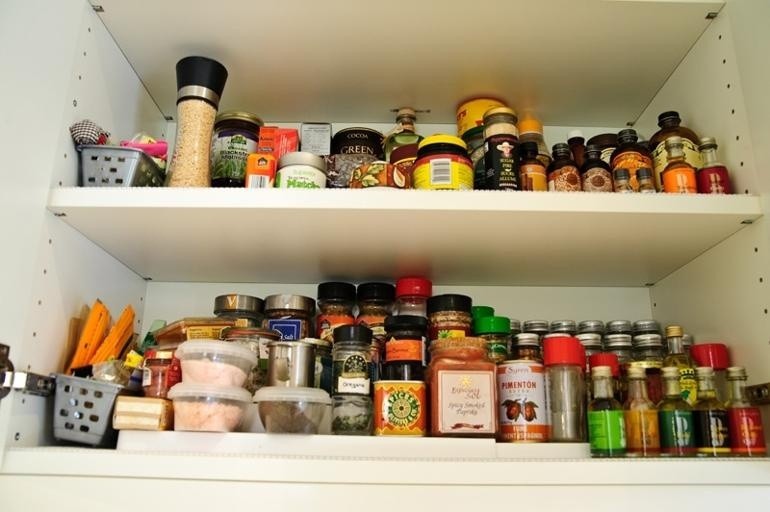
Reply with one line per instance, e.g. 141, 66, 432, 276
141, 350, 176, 398
207, 111, 264, 186
331, 127, 384, 157
171, 278, 511, 438
494, 359, 555, 443
409, 96, 523, 191
350, 161, 411, 188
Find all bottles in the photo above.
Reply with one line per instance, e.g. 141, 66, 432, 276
164, 56, 228, 187
382, 105, 423, 159
379, 275, 476, 380
482, 106, 733, 195
546, 318, 769, 459
475, 303, 547, 359
313, 272, 394, 437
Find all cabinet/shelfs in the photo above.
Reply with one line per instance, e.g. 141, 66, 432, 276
4, 186, 769, 487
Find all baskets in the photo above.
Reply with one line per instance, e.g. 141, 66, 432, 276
52, 372, 127, 447
78, 143, 167, 187
